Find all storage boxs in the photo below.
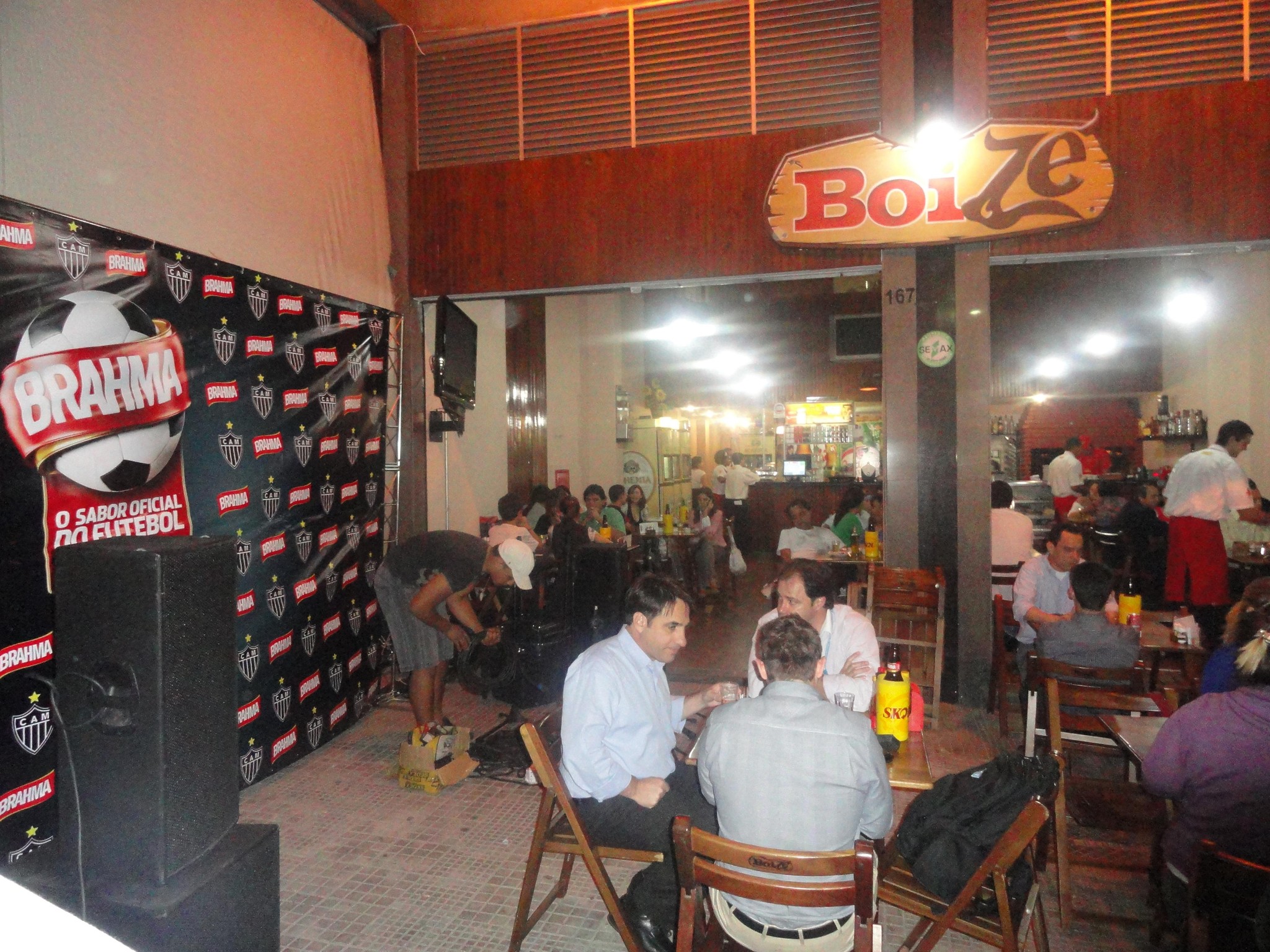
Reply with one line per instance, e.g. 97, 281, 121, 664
399, 723, 480, 794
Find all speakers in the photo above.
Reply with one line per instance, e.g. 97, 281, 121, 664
17, 822, 283, 952
54, 531, 241, 884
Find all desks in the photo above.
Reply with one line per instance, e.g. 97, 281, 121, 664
747, 478, 882, 553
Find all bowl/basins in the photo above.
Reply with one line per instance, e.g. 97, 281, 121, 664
831, 553, 847, 560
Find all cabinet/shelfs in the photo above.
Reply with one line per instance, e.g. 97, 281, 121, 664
1009, 481, 1057, 541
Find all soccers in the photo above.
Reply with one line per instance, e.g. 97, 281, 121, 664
14, 290, 186, 494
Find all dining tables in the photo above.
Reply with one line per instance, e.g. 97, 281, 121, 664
813, 546, 884, 609
685, 681, 935, 858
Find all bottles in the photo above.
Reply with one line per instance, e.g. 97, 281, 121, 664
865, 517, 879, 557
662, 503, 673, 536
679, 499, 687, 523
850, 527, 859, 558
599, 514, 611, 543
1118, 554, 1142, 626
1138, 408, 1204, 436
990, 413, 1017, 438
875, 642, 912, 742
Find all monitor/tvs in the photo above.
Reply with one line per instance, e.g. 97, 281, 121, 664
435, 294, 478, 410
829, 313, 882, 361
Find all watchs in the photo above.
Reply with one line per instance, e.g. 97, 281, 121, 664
1118, 482, 1170, 576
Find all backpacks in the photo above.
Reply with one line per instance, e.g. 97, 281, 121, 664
896, 746, 1059, 929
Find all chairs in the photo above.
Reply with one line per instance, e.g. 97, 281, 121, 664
875, 747, 1053, 952
502, 517, 740, 648
508, 695, 673, 952
865, 564, 946, 734
990, 520, 1270, 952
670, 816, 877, 951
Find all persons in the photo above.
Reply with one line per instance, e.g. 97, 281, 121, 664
1219, 482, 1269, 600
372, 530, 535, 732
991, 480, 1036, 604
479, 456, 910, 584
1160, 419, 1270, 652
688, 610, 898, 952
1046, 435, 1084, 526
1073, 434, 1113, 474
1198, 577, 1270, 700
1066, 479, 1102, 524
1081, 479, 1127, 525
1137, 595, 1270, 952
552, 572, 748, 951
1035, 560, 1140, 724
1011, 519, 1121, 702
745, 556, 881, 712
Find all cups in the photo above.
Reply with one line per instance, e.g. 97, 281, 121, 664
831, 541, 840, 552
834, 692, 856, 711
719, 679, 738, 706
677, 519, 683, 532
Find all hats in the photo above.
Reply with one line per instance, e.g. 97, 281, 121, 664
499, 537, 535, 592
